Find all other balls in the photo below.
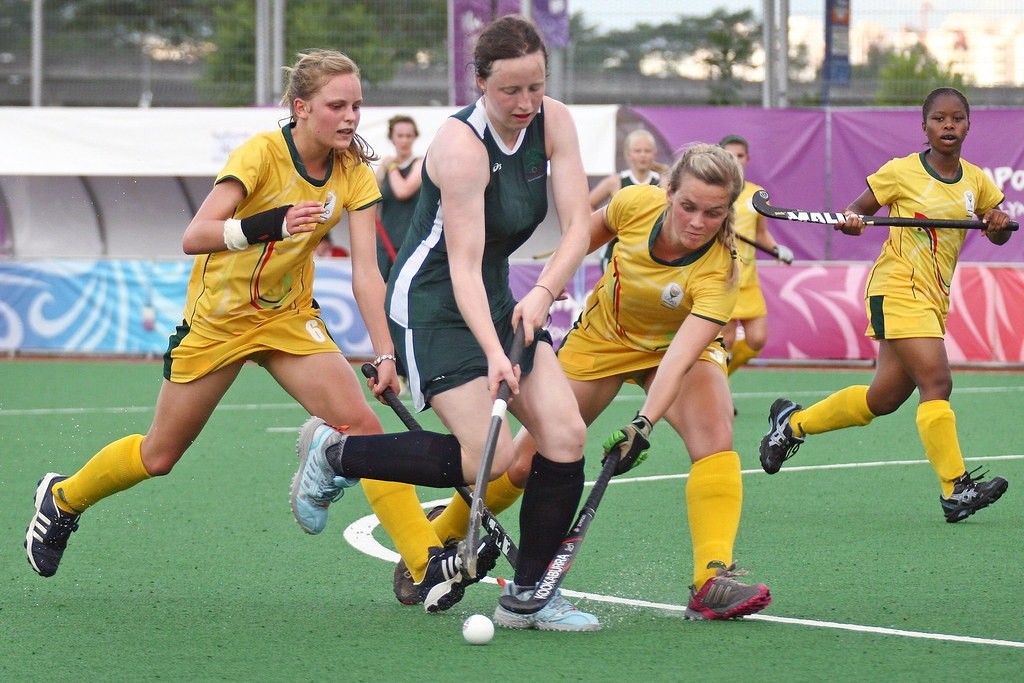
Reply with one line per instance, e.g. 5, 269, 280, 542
461, 613, 495, 645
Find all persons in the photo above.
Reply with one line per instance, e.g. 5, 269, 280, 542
372, 115, 425, 396
289, 17, 600, 634
22, 47, 500, 612
393, 140, 778, 621
588, 122, 665, 276
758, 85, 1012, 525
708, 138, 796, 416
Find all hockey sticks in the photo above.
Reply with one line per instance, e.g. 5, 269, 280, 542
459, 317, 528, 581
361, 360, 520, 571
735, 233, 792, 266
751, 189, 1020, 231
497, 446, 621, 616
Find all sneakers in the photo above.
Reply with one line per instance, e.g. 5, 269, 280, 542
291, 416, 366, 535
23, 471, 83, 579
683, 556, 770, 620
759, 400, 808, 475
939, 466, 1009, 523
494, 578, 600, 632
411, 535, 500, 613
394, 503, 446, 605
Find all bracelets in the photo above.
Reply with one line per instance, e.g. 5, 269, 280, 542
373, 355, 397, 366
386, 163, 397, 172
534, 285, 556, 304
